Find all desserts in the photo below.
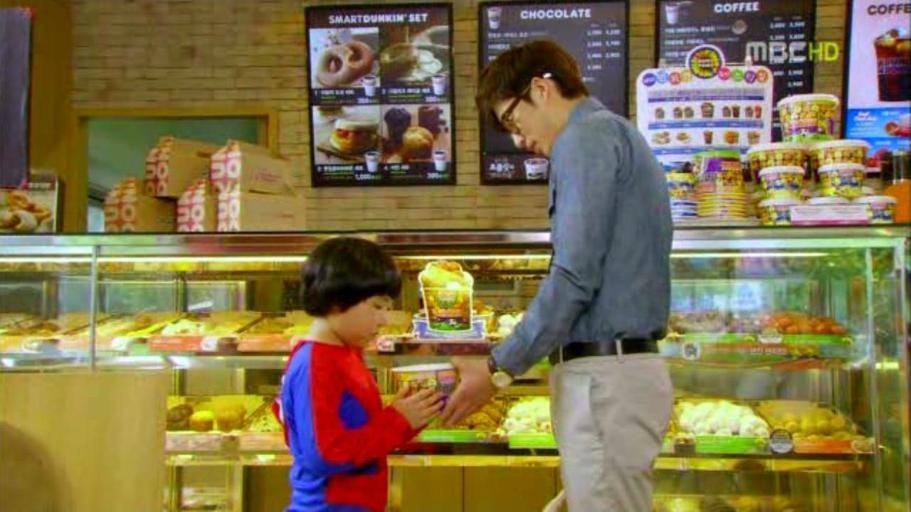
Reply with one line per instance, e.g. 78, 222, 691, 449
0, 299, 643, 360
166, 398, 858, 437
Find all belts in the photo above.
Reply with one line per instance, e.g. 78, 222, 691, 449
547, 339, 660, 365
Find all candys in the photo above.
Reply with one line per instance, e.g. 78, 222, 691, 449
749, 99, 894, 228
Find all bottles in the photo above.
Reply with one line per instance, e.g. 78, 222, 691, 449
884, 148, 911, 186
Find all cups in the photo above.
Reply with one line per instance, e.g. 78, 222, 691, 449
362, 75, 377, 99
488, 6, 502, 34
365, 151, 380, 173
875, 38, 911, 102
662, 149, 749, 223
431, 74, 446, 97
654, 103, 762, 148
433, 151, 448, 172
749, 92, 896, 224
523, 157, 548, 179
665, 5, 681, 25
424, 285, 473, 330
388, 360, 459, 406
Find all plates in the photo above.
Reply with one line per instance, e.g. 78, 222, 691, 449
32, 207, 50, 221
314, 62, 381, 89
0, 212, 25, 232
389, 54, 443, 81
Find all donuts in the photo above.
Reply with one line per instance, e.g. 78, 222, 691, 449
317, 45, 353, 86
343, 41, 372, 77
378, 42, 417, 72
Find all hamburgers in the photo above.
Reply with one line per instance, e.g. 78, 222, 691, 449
329, 115, 377, 154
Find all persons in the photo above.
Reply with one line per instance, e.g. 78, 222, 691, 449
441, 40, 679, 512
271, 235, 448, 512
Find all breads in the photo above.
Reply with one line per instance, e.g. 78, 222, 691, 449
402, 126, 432, 158
29, 207, 50, 224
667, 306, 844, 336
7, 190, 35, 214
4, 210, 37, 234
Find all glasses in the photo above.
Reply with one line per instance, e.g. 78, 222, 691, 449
500, 73, 552, 132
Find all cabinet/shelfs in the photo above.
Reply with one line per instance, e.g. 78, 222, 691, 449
1, 238, 910, 511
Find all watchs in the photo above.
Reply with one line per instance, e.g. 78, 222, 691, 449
487, 357, 514, 390
